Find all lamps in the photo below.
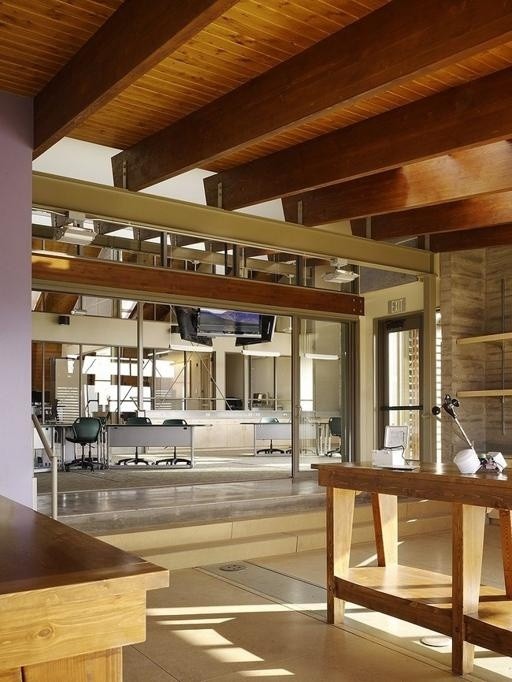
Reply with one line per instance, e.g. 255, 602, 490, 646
59, 315, 70, 325
171, 325, 180, 334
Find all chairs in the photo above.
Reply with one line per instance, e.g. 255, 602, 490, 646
116, 416, 155, 466
324, 415, 343, 456
152, 417, 192, 467
63, 415, 107, 473
286, 417, 315, 453
256, 415, 283, 453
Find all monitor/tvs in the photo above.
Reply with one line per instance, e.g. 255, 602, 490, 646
171, 306, 277, 346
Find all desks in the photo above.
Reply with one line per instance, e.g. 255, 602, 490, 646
41, 422, 105, 470
238, 421, 333, 456
105, 422, 208, 466
309, 461, 511, 677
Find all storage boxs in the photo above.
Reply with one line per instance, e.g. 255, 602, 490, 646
368, 424, 410, 471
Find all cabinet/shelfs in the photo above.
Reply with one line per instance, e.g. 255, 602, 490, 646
229, 421, 265, 452
150, 420, 194, 452
1, 492, 170, 682
195, 420, 229, 451
264, 422, 293, 448
453, 331, 512, 400
